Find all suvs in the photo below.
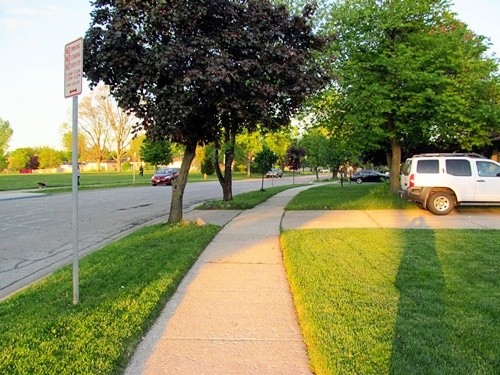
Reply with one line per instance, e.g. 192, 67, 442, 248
397, 153, 500, 215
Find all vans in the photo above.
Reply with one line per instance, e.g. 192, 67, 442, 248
151, 167, 180, 187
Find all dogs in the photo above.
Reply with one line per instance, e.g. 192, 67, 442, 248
37, 182, 47, 189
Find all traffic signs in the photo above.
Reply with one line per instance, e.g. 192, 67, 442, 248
64, 36, 84, 99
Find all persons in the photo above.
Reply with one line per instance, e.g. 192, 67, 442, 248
77, 166, 80, 185
139, 165, 143, 176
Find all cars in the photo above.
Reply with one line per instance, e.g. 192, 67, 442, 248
266, 170, 282, 179
350, 170, 390, 184
